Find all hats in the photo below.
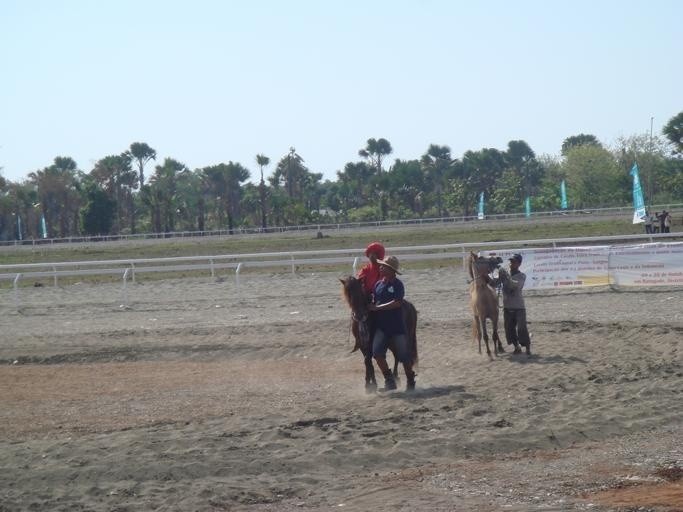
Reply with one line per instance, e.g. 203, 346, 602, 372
376, 255, 403, 275
508, 254, 522, 265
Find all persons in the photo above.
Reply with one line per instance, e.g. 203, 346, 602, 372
365, 255, 417, 392
656, 210, 668, 233
640, 211, 653, 234
355, 241, 385, 300
487, 252, 532, 355
652, 212, 659, 234
665, 213, 672, 233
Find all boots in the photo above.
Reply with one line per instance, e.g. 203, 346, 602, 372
403, 372, 416, 392
377, 369, 397, 392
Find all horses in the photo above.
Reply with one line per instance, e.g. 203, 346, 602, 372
464, 250, 506, 363
337, 272, 418, 396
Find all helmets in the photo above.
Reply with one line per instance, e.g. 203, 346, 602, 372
365, 242, 385, 260
